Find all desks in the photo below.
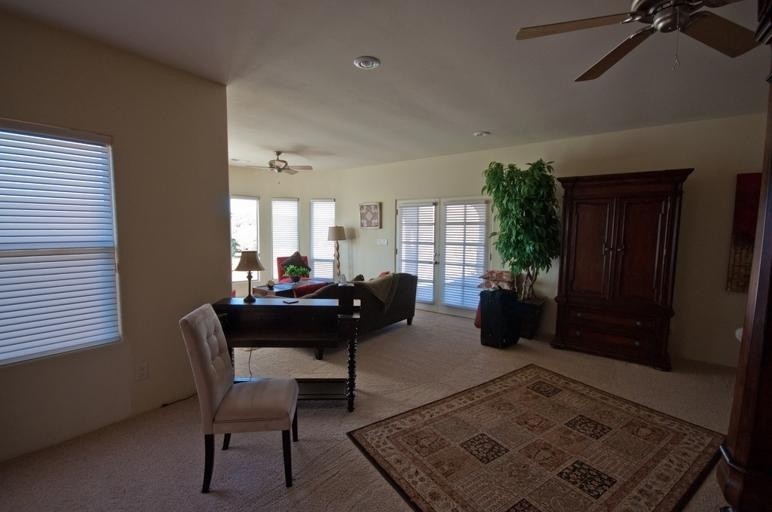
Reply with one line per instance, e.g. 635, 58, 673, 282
207, 296, 363, 413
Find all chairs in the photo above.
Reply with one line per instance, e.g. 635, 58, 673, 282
179, 302, 303, 495
276, 255, 308, 283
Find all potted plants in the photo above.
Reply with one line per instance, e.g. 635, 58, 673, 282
481, 158, 562, 340
284, 264, 309, 283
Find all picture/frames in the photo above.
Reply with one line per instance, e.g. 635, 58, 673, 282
359, 202, 381, 229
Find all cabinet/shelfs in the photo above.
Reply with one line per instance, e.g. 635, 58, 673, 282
556, 166, 696, 372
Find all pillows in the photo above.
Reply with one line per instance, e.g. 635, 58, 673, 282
280, 251, 311, 282
293, 274, 367, 298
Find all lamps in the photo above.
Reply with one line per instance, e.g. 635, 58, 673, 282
327, 225, 346, 284
236, 250, 266, 302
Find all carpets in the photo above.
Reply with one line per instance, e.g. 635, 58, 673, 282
345, 362, 728, 511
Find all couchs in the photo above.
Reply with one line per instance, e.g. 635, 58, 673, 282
290, 273, 418, 360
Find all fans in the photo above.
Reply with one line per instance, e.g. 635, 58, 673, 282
513, 2, 761, 84
247, 151, 313, 176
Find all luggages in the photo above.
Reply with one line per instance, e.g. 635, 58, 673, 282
480, 288, 520, 348
